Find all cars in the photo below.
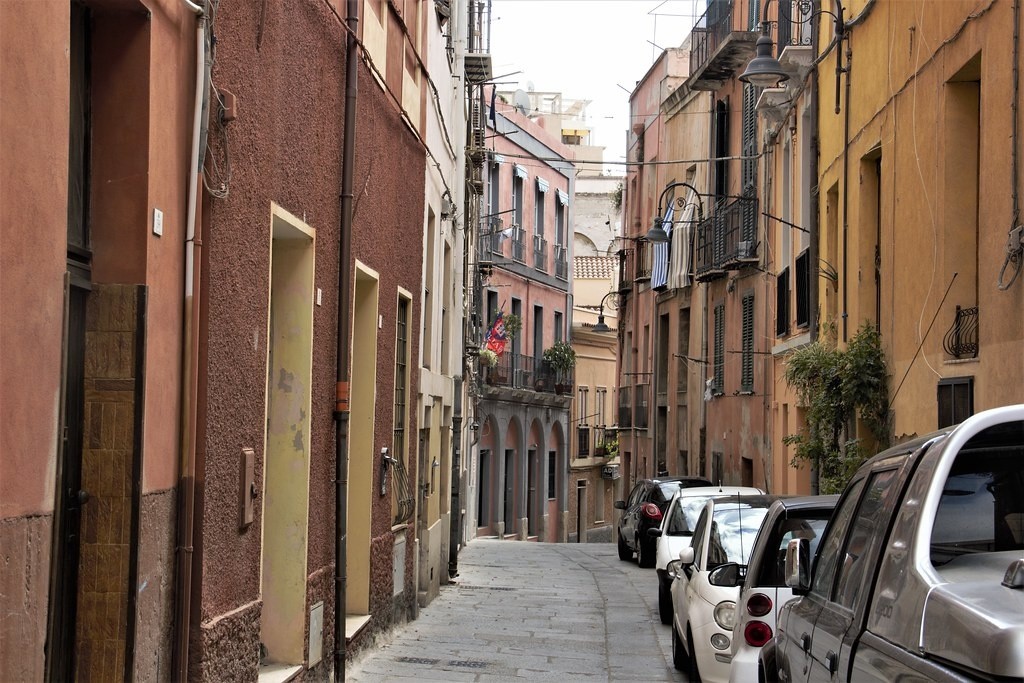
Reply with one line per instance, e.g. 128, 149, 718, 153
670, 496, 798, 683
613, 476, 714, 568
645, 486, 770, 623
731, 495, 846, 682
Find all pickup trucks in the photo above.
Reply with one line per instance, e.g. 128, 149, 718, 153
758, 400, 1024, 683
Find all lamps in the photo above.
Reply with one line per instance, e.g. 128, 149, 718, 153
642, 182, 709, 258
591, 292, 625, 332
736, 1, 851, 115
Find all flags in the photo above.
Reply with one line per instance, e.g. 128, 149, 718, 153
481, 310, 506, 358
